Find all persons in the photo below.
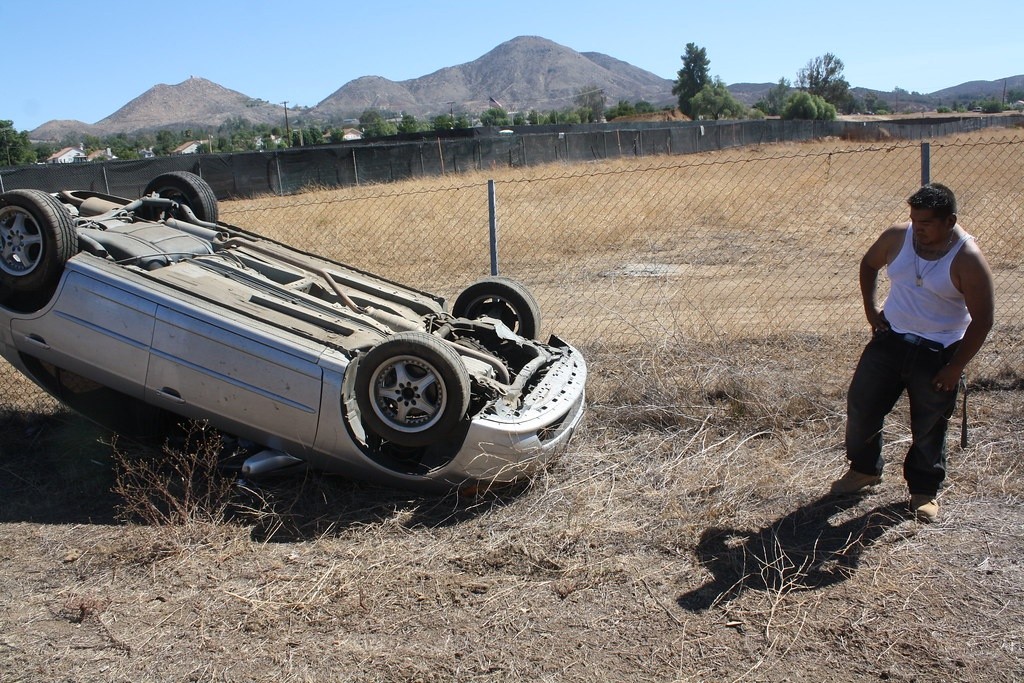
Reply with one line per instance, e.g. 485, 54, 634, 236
830, 183, 995, 522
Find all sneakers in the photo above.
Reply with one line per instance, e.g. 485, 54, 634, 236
910, 495, 938, 520
831, 468, 880, 494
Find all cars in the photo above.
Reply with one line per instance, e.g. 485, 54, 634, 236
0, 171, 587, 496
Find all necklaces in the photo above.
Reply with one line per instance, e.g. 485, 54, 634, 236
914, 230, 954, 286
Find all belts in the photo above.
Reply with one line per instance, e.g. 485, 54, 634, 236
888, 329, 944, 358
